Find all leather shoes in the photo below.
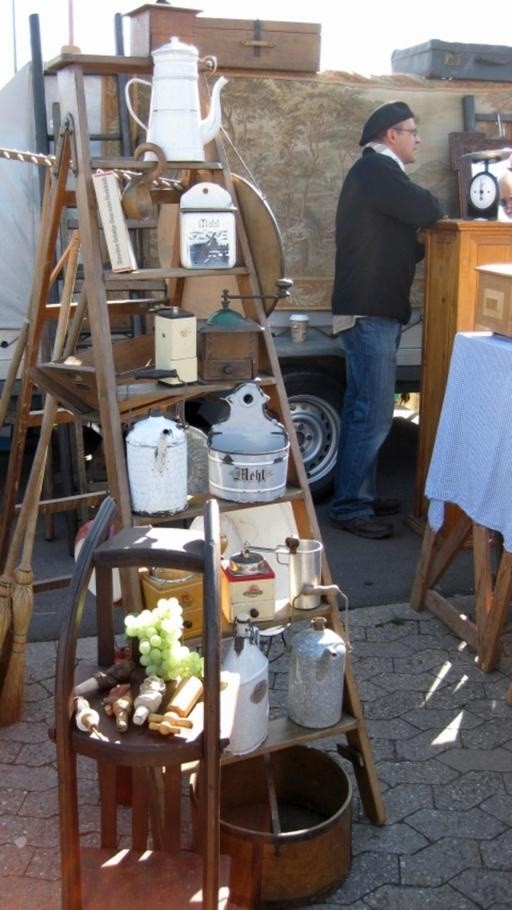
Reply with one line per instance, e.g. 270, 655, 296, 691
373, 498, 402, 515
329, 515, 395, 538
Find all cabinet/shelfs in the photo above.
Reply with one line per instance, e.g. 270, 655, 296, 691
52, 497, 227, 910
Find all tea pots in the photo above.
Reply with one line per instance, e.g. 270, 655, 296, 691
122, 367, 190, 525
125, 33, 231, 172
281, 582, 357, 730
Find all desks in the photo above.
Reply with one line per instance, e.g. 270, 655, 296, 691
404, 329, 512, 673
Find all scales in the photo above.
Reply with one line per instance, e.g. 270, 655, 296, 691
459, 149, 512, 220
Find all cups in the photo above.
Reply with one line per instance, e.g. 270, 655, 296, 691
288, 313, 309, 344
275, 538, 326, 609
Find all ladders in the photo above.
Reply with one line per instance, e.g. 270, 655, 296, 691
0, 51, 385, 858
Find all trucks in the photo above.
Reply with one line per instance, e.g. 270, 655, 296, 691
0, 52, 511, 507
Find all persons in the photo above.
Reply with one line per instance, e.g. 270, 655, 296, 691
498, 172, 512, 220
328, 101, 445, 541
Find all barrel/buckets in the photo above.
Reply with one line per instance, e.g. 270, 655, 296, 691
189, 741, 352, 907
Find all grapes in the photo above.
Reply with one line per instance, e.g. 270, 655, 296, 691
125, 595, 203, 685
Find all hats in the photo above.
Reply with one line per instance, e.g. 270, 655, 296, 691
358, 102, 414, 146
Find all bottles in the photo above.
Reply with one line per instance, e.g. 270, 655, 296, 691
224, 613, 270, 760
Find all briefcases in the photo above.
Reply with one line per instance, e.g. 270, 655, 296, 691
391, 39, 512, 84
194, 17, 321, 72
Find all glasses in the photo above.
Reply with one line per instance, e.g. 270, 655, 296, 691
393, 127, 417, 136
